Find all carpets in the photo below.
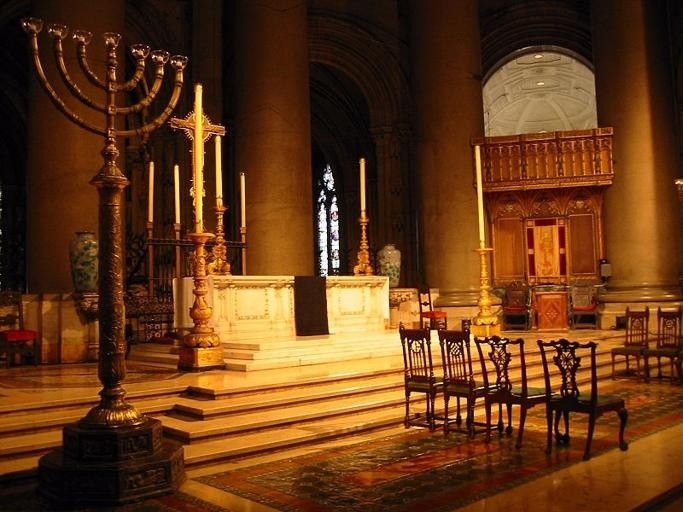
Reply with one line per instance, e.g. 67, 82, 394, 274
193, 379, 683, 512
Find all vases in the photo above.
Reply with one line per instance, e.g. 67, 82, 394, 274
376, 243, 402, 287
68, 230, 99, 292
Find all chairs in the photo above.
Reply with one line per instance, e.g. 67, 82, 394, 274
568, 279, 600, 329
501, 281, 530, 331
611, 305, 649, 383
438, 330, 504, 438
537, 338, 629, 461
643, 306, 683, 385
473, 335, 564, 449
398, 321, 461, 433
418, 288, 448, 330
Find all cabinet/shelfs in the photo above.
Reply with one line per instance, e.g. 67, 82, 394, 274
532, 292, 570, 331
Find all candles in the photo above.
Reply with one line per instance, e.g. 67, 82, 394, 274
476, 146, 485, 248
174, 165, 181, 223
360, 159, 366, 219
148, 162, 154, 222
195, 85, 204, 233
241, 173, 246, 227
216, 136, 222, 207
